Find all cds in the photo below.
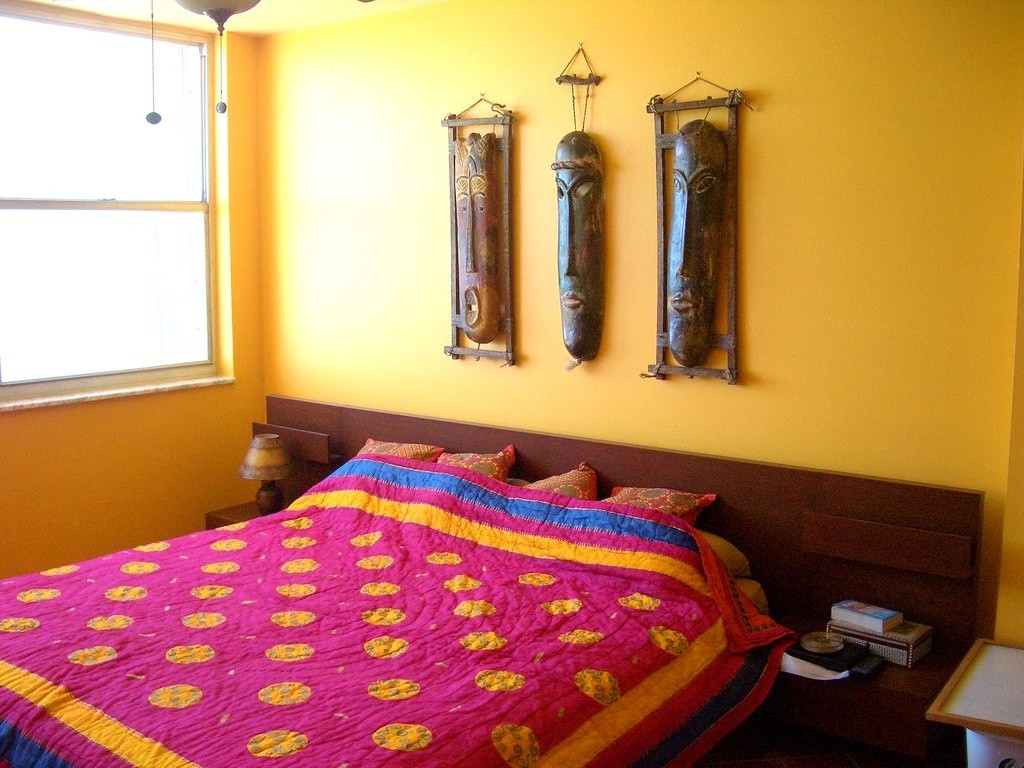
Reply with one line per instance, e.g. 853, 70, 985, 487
801, 632, 842, 648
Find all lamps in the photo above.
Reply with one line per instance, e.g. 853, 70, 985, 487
239, 434, 294, 516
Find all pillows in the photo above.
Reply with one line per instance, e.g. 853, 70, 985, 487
600, 487, 717, 526
357, 438, 446, 464
522, 461, 597, 502
437, 443, 515, 484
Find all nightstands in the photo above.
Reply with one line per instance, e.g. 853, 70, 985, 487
781, 614, 961, 760
205, 502, 263, 531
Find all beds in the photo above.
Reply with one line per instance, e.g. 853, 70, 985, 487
0, 395, 771, 768
